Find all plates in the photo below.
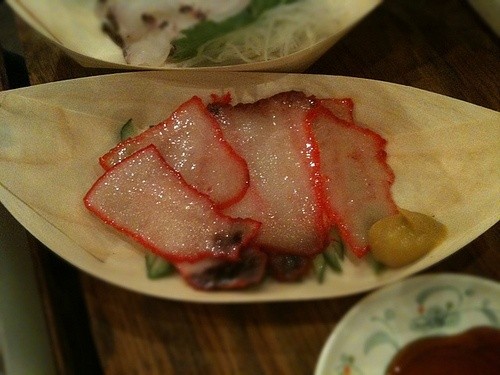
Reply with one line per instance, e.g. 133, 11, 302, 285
0, 71, 500, 304
4, 0, 383, 72
314, 273, 500, 375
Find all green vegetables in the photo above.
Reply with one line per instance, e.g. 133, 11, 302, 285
171, 0, 297, 64
